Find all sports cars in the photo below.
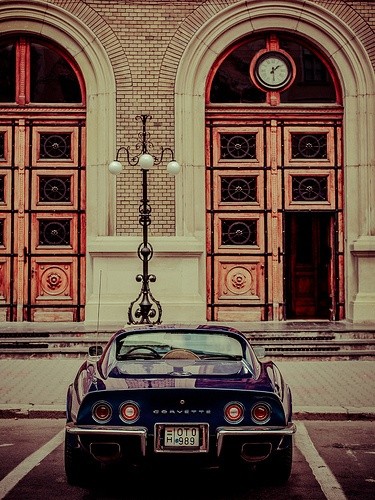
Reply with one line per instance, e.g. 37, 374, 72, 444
65, 324, 295, 483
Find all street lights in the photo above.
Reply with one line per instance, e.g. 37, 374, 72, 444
108, 115, 184, 326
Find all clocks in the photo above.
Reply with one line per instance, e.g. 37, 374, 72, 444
254, 50, 294, 91
